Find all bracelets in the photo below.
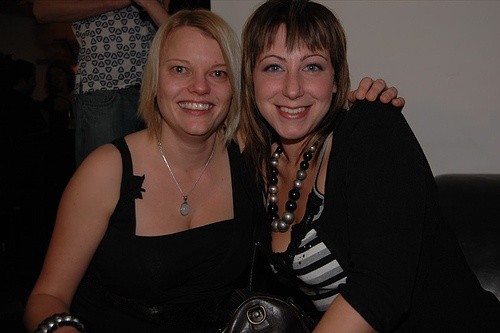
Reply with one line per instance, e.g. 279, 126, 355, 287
33, 312, 87, 332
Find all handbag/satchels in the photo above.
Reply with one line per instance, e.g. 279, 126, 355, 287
227, 293, 314, 333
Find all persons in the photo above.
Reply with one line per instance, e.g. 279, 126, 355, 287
25, 9, 406, 332
1, 0, 172, 171
231, 0, 500, 333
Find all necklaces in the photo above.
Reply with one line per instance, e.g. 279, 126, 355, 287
158, 123, 217, 216
267, 135, 328, 232
